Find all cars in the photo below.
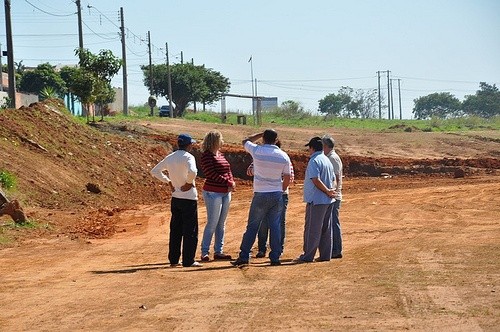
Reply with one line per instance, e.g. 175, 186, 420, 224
159, 106, 174, 117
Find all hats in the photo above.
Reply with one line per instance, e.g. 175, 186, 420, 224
179, 135, 196, 145
305, 137, 322, 146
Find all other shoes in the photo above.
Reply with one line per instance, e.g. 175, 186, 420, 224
332, 255, 342, 258
201, 254, 209, 261
214, 254, 231, 260
230, 258, 249, 265
171, 262, 182, 266
256, 253, 265, 258
300, 255, 313, 262
315, 257, 330, 262
191, 262, 203, 267
271, 261, 281, 266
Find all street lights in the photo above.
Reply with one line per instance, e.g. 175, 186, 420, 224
248, 56, 254, 115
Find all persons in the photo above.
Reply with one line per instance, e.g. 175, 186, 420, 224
151, 133, 204, 267
294, 137, 338, 262
230, 128, 291, 266
200, 130, 236, 261
321, 134, 343, 258
247, 140, 295, 258
